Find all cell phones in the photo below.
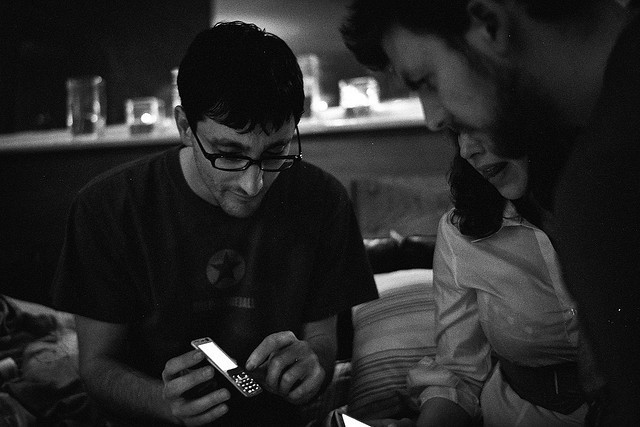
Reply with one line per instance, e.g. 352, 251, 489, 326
191, 336, 264, 398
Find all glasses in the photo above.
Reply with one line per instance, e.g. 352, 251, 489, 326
185, 109, 303, 172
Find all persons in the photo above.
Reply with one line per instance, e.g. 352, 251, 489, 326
334, 0, 638, 427
414, 121, 593, 426
48, 20, 378, 425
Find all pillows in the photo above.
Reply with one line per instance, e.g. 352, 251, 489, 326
350, 279, 452, 415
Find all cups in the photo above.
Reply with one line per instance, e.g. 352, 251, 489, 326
66, 74, 106, 137
338, 75, 380, 119
122, 94, 159, 134
